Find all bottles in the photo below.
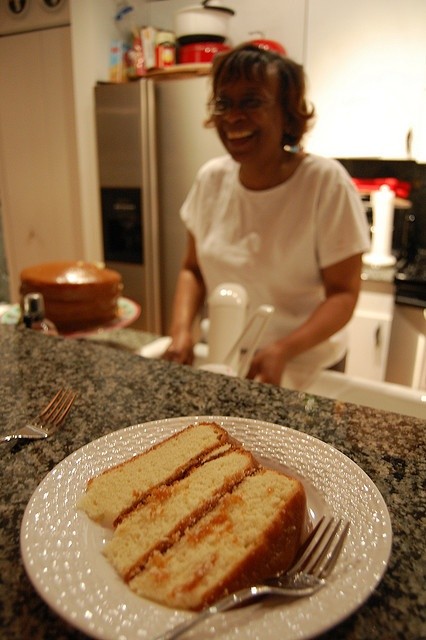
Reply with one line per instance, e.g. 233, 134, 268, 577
16, 291, 60, 333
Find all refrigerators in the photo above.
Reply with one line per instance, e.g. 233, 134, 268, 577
94, 75, 229, 344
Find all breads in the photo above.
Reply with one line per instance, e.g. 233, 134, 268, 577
102, 450, 259, 584
132, 469, 306, 611
81, 422, 240, 527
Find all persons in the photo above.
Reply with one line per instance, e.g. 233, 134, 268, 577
157, 39, 372, 387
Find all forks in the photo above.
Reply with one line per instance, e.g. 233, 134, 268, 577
0, 385, 75, 444
155, 515, 351, 639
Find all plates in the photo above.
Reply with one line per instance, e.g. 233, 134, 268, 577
59, 295, 142, 338
20, 414, 393, 640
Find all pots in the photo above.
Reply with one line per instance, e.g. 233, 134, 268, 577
175, 0, 236, 46
